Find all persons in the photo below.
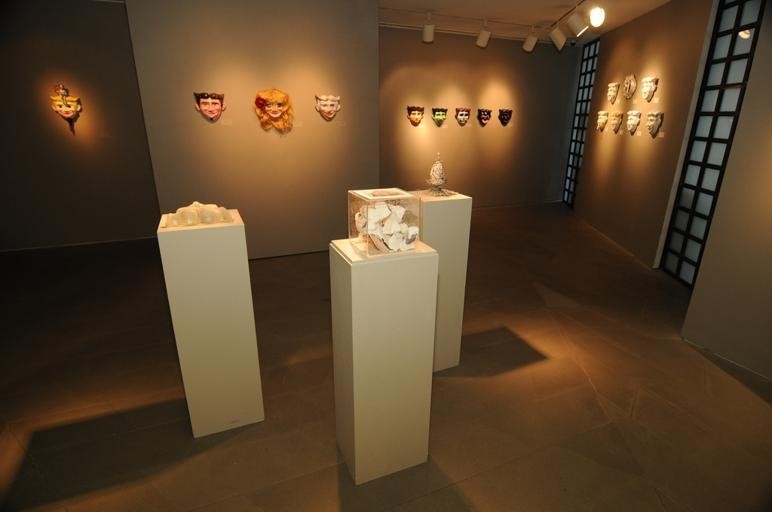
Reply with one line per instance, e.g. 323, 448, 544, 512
454, 107, 471, 127
313, 94, 342, 120
431, 107, 449, 126
476, 108, 493, 126
254, 87, 296, 136
190, 92, 226, 122
596, 75, 664, 139
498, 109, 513, 128
406, 105, 427, 125
51, 95, 83, 124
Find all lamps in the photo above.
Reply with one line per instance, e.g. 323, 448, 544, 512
421, 5, 606, 53
